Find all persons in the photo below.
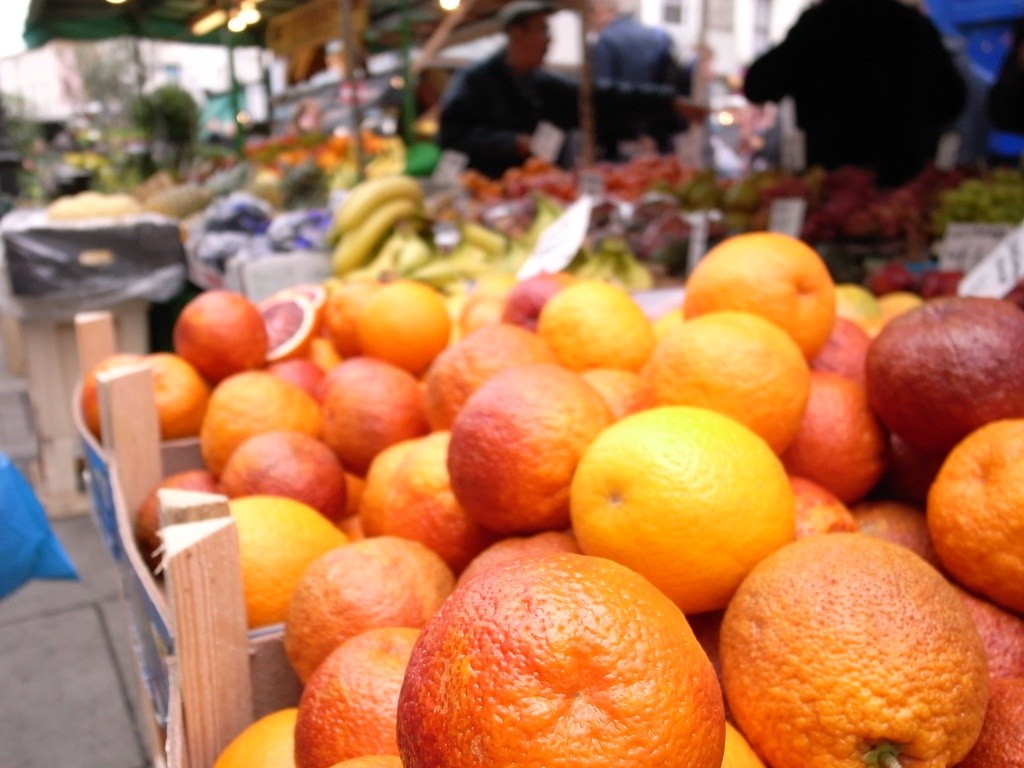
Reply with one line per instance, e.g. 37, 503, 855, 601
433, 0, 1024, 190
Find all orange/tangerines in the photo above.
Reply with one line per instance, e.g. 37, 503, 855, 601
77, 230, 1024, 768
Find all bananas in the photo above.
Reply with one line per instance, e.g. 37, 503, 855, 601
331, 172, 653, 293
